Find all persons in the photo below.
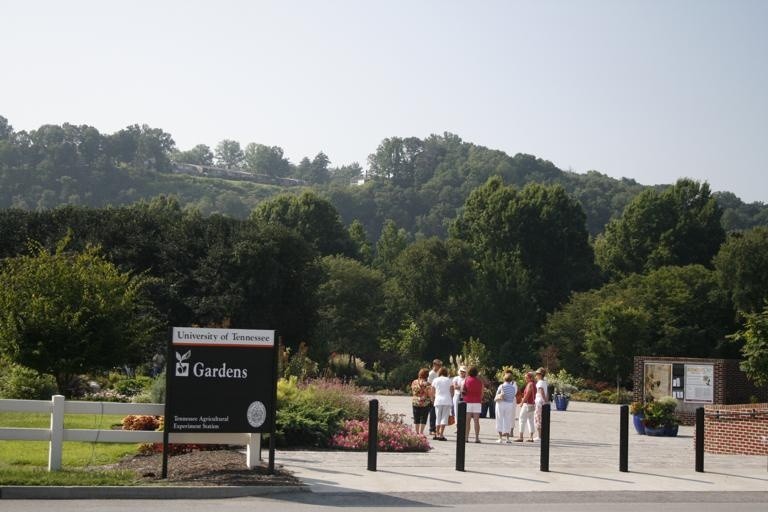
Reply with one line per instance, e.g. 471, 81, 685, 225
411, 360, 551, 445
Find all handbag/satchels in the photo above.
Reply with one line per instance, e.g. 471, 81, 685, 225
527, 405, 536, 412
516, 385, 522, 404
493, 385, 504, 401
448, 415, 455, 425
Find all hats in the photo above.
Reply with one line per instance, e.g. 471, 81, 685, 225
433, 359, 442, 365
535, 367, 546, 376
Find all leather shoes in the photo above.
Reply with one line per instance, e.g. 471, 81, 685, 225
433, 436, 439, 440
526, 439, 534, 442
514, 439, 523, 442
439, 437, 447, 441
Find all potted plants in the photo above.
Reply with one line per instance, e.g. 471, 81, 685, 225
548, 368, 582, 412
629, 394, 652, 434
642, 398, 678, 436
